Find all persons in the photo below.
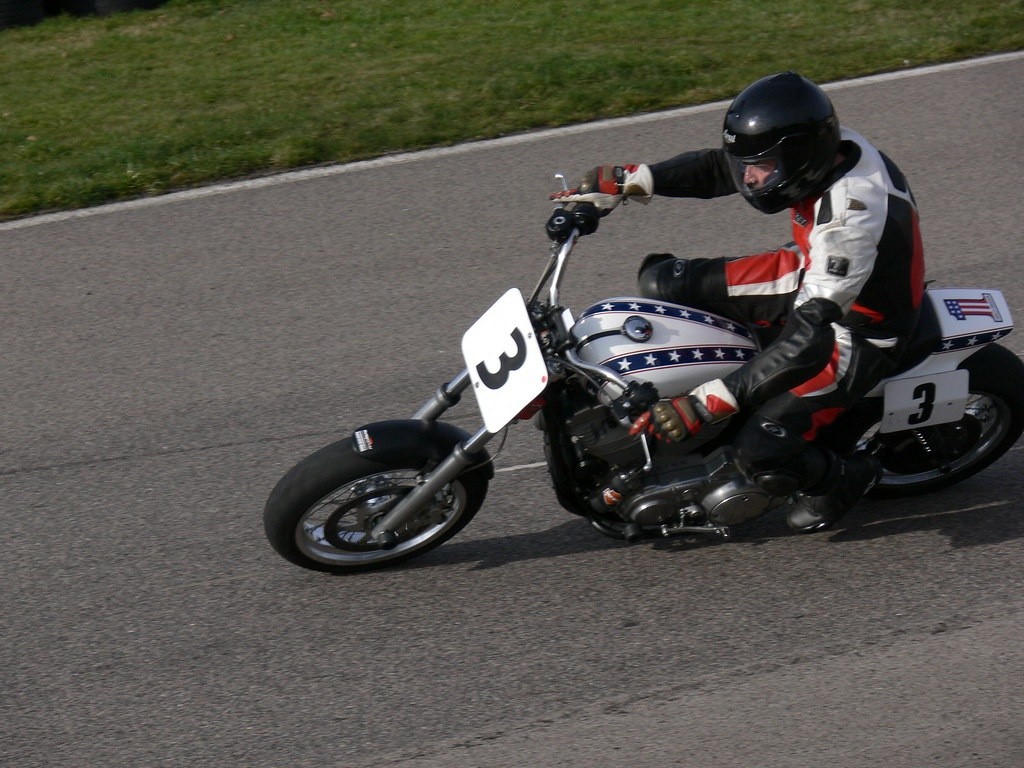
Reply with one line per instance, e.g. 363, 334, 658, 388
558, 70, 927, 533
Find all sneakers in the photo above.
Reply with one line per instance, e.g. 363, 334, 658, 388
786, 456, 881, 534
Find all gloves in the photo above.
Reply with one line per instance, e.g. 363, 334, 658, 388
629, 377, 741, 447
549, 162, 655, 218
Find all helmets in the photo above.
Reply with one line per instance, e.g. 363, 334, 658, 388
722, 71, 841, 215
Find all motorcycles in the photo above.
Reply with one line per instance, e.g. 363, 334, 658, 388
262, 173, 1023, 575
